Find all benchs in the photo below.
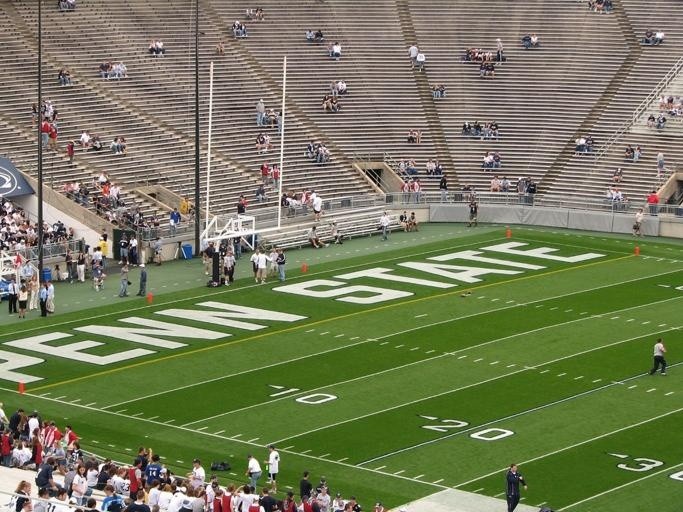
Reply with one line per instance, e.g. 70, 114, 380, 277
0, 439, 259, 512
0, 0, 683, 251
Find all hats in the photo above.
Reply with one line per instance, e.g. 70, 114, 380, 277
139, 264, 145, 267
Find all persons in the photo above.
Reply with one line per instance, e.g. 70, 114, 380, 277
231, 6, 449, 249
33, 100, 128, 166
1, 171, 160, 321
58, 69, 71, 86
647, 338, 673, 379
0, 400, 386, 512
501, 461, 531, 511
170, 196, 288, 287
643, 28, 664, 46
99, 61, 128, 82
587, 0, 614, 14
57, 0, 76, 12
148, 39, 166, 58
458, 33, 541, 227
575, 95, 682, 238
